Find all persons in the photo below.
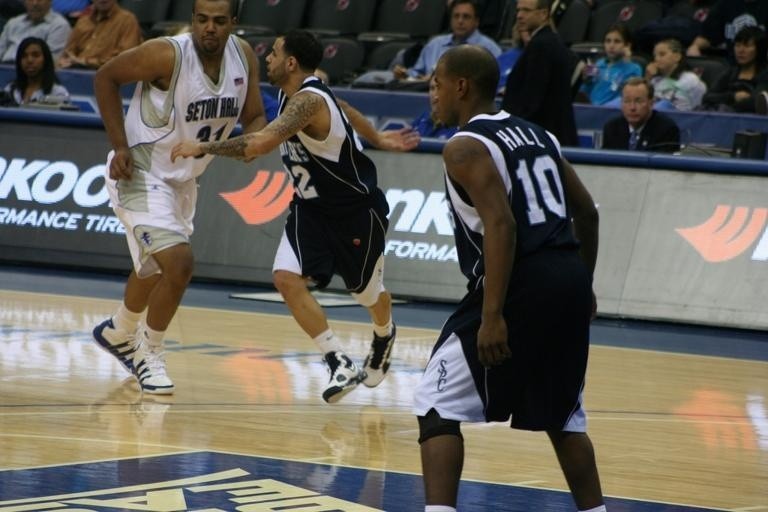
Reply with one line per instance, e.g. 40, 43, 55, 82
0, 1, 72, 67
395, 1, 504, 81
601, 77, 681, 153
0, 36, 70, 111
170, 30, 421, 405
501, 1, 578, 149
578, 2, 766, 116
55, 0, 143, 71
410, 44, 610, 512
91, 0, 267, 396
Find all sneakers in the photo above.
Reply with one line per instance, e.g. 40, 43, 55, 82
321, 349, 369, 405
129, 330, 176, 397
89, 313, 143, 375
361, 321, 397, 389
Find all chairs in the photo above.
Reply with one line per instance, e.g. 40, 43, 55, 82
0, 1, 768, 162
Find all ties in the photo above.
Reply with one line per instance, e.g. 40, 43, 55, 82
628, 130, 638, 153
